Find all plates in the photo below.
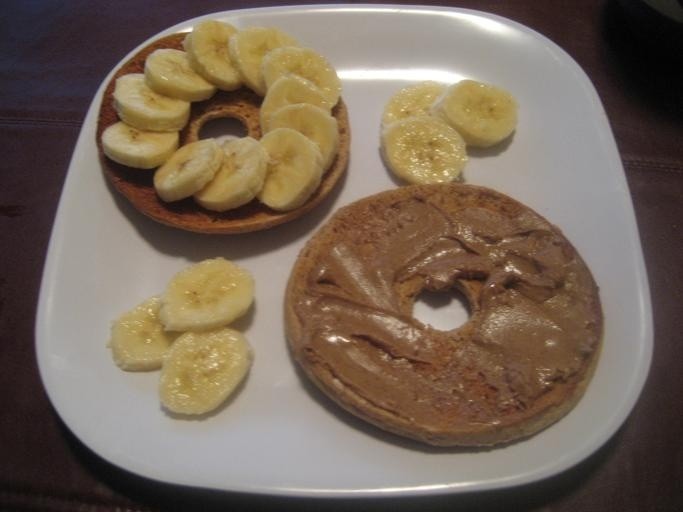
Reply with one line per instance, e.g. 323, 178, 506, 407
30, 4, 652, 500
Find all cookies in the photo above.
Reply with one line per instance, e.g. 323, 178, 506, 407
283, 184, 603, 448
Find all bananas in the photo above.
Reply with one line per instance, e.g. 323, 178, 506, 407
110, 256, 256, 416
101, 18, 342, 213
379, 80, 518, 186
